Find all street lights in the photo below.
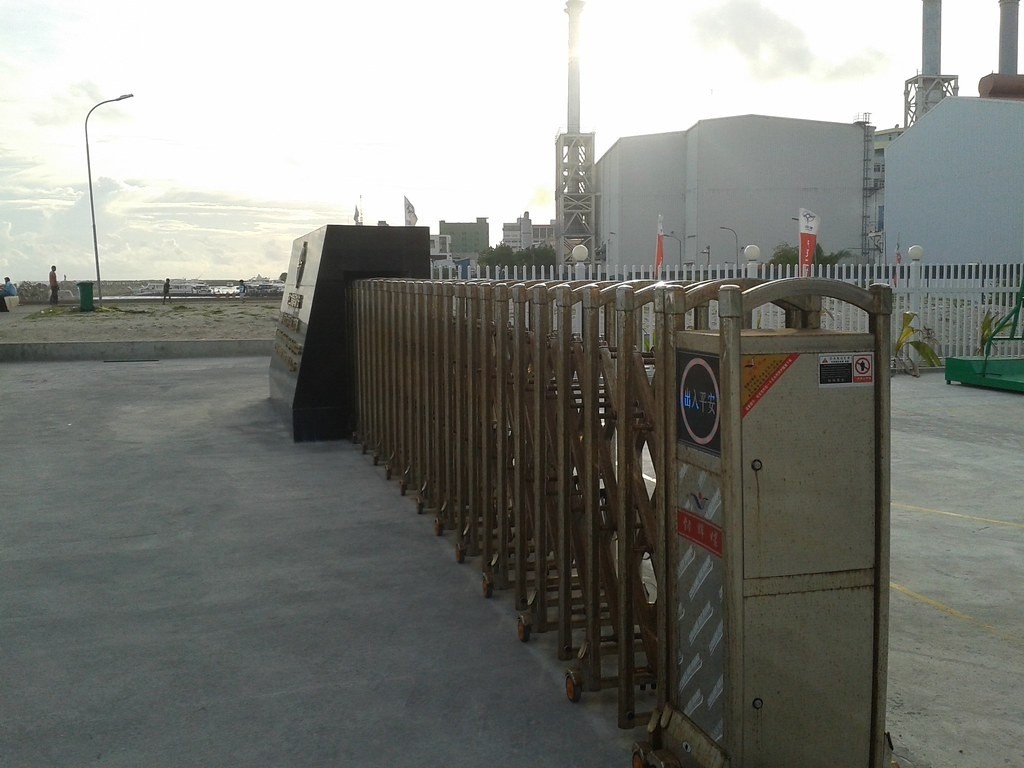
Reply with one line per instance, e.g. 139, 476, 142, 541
719, 225, 740, 272
662, 234, 682, 274
84, 94, 136, 313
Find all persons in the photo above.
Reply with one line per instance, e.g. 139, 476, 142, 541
240, 280, 246, 303
0, 277, 17, 300
49, 266, 60, 305
163, 278, 172, 305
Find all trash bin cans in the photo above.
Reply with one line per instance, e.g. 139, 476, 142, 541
77, 281, 95, 311
